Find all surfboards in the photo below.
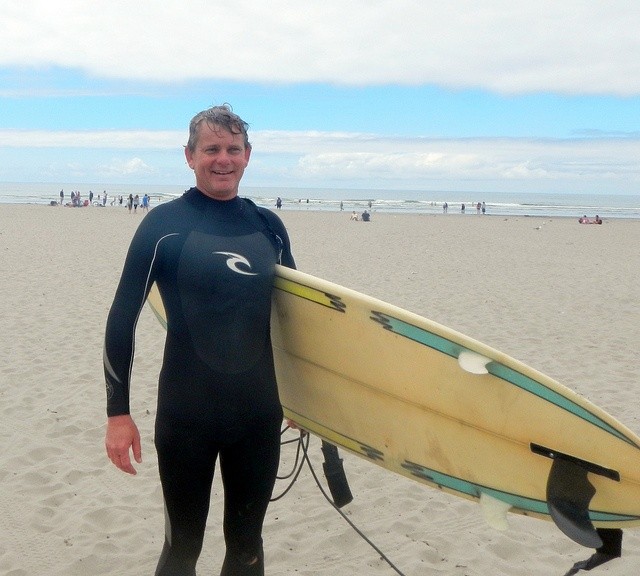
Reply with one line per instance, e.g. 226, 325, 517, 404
145, 263, 640, 532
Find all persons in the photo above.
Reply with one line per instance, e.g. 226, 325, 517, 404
89, 190, 93, 205
593, 215, 602, 224
143, 193, 149, 212
69, 190, 82, 207
579, 215, 592, 224
362, 210, 370, 221
102, 106, 305, 576
60, 189, 64, 205
477, 202, 481, 214
350, 211, 359, 222
481, 202, 486, 214
126, 193, 133, 214
133, 195, 140, 214
